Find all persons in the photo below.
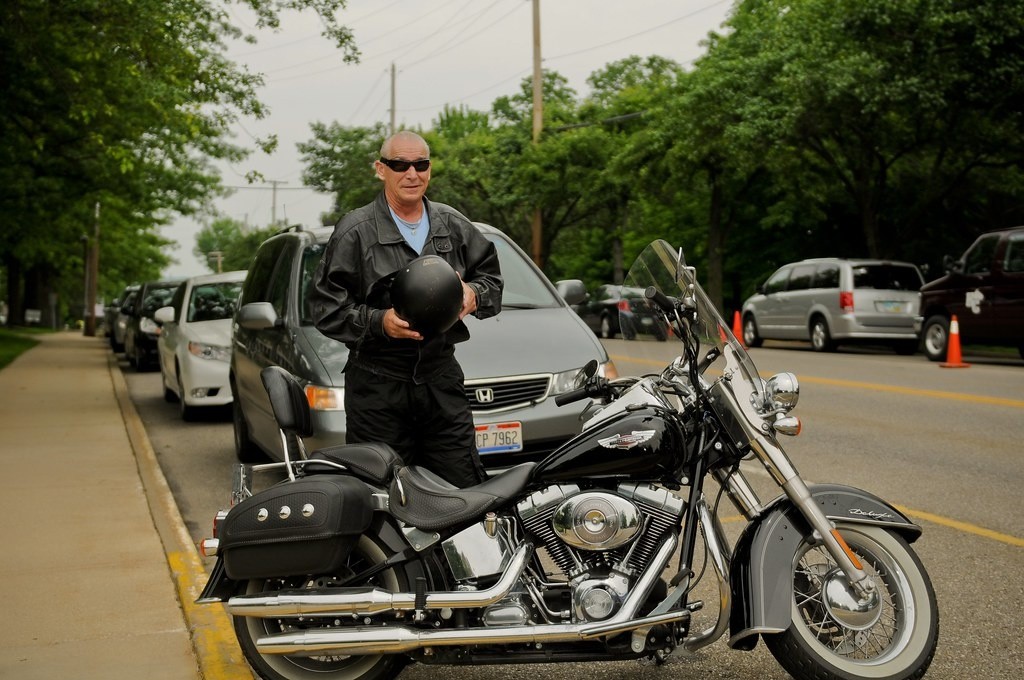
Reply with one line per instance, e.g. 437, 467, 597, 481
307, 130, 504, 489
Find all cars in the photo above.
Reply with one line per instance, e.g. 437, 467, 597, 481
569, 284, 668, 342
103, 285, 142, 353
120, 280, 181, 373
154, 270, 249, 423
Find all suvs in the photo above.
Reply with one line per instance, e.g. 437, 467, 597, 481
228, 221, 621, 479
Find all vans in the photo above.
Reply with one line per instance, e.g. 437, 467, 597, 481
913, 227, 1024, 362
741, 257, 925, 353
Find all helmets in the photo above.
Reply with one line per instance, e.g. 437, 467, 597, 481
389, 254, 463, 339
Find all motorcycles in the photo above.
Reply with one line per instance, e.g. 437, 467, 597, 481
192, 239, 940, 680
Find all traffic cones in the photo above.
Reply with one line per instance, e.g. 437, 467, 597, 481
939, 314, 972, 367
732, 311, 749, 351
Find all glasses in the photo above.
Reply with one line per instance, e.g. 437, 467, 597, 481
380, 156, 430, 172
